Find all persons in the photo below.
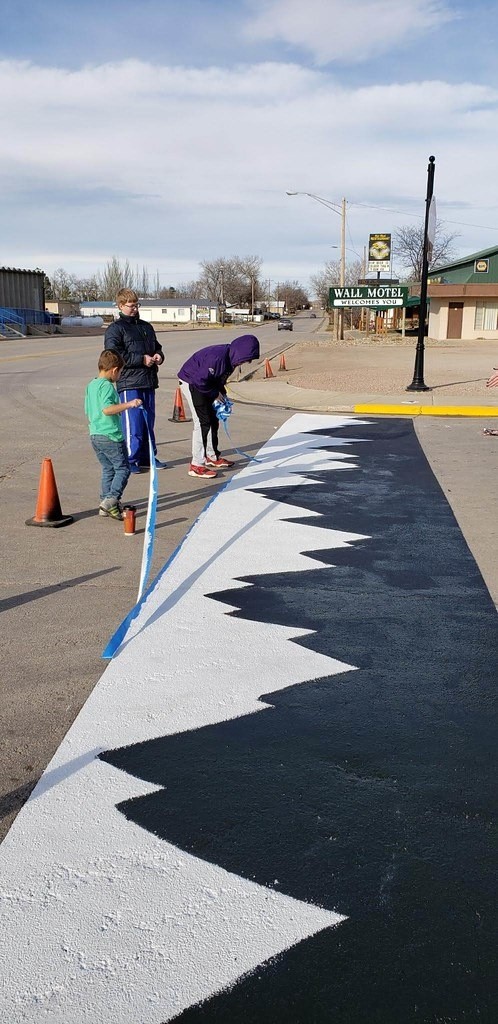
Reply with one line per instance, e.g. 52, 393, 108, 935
177, 334, 260, 479
104, 287, 168, 474
84, 349, 144, 521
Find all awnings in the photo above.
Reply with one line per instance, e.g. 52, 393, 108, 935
402, 296, 430, 308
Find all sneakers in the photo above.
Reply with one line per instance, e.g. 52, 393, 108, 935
205, 457, 235, 467
99, 501, 124, 517
138, 458, 167, 470
188, 464, 218, 478
99, 498, 122, 520
129, 464, 141, 473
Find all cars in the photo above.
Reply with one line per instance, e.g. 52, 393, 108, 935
277, 318, 293, 331
310, 313, 316, 319
262, 312, 280, 321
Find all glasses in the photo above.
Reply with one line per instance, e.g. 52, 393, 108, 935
122, 302, 141, 309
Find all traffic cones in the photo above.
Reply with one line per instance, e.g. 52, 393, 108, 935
278, 356, 289, 372
168, 388, 193, 423
263, 358, 277, 379
25, 457, 76, 529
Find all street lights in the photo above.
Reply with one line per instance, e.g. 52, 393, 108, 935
329, 243, 367, 329
286, 188, 346, 340
